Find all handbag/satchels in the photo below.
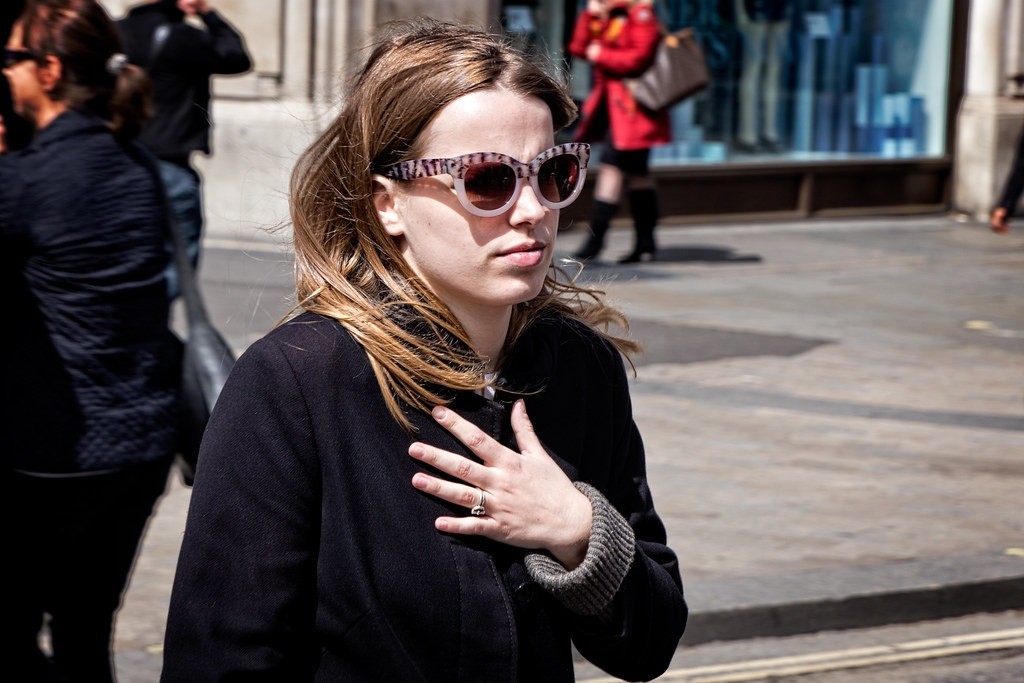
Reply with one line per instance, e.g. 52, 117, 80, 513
138, 146, 236, 490
619, 8, 710, 115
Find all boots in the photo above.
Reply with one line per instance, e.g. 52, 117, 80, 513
574, 197, 617, 261
615, 187, 659, 264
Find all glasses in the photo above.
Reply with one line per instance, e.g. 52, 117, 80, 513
0, 47, 41, 71
385, 143, 591, 216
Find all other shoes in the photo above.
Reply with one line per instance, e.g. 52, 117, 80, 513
992, 213, 1010, 232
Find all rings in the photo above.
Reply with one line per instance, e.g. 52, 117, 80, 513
472, 487, 486, 516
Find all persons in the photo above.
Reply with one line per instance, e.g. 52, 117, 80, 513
569, 0, 677, 265
162, 0, 693, 683
0, 0, 239, 683
990, 126, 1024, 232
